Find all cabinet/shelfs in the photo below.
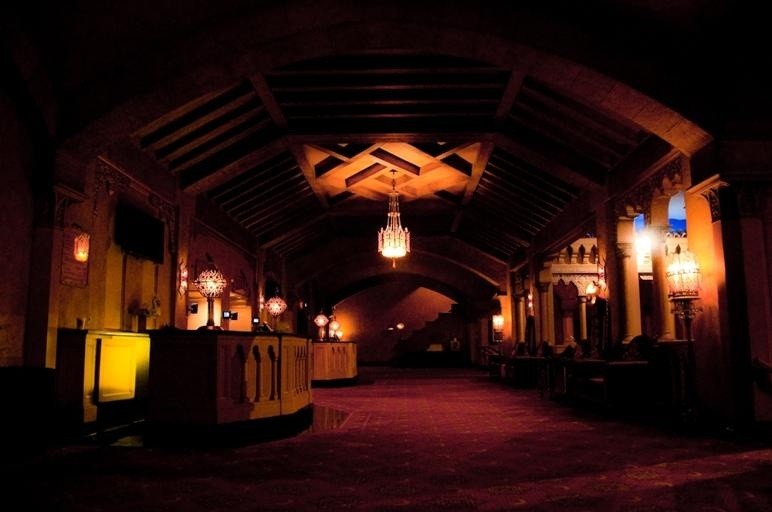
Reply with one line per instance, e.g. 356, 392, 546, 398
60, 328, 151, 423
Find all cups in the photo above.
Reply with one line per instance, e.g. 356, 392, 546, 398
76, 317, 85, 330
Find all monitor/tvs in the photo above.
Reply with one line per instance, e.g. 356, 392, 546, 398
114, 199, 163, 263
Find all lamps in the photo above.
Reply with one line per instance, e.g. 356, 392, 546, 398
377, 169, 412, 260
